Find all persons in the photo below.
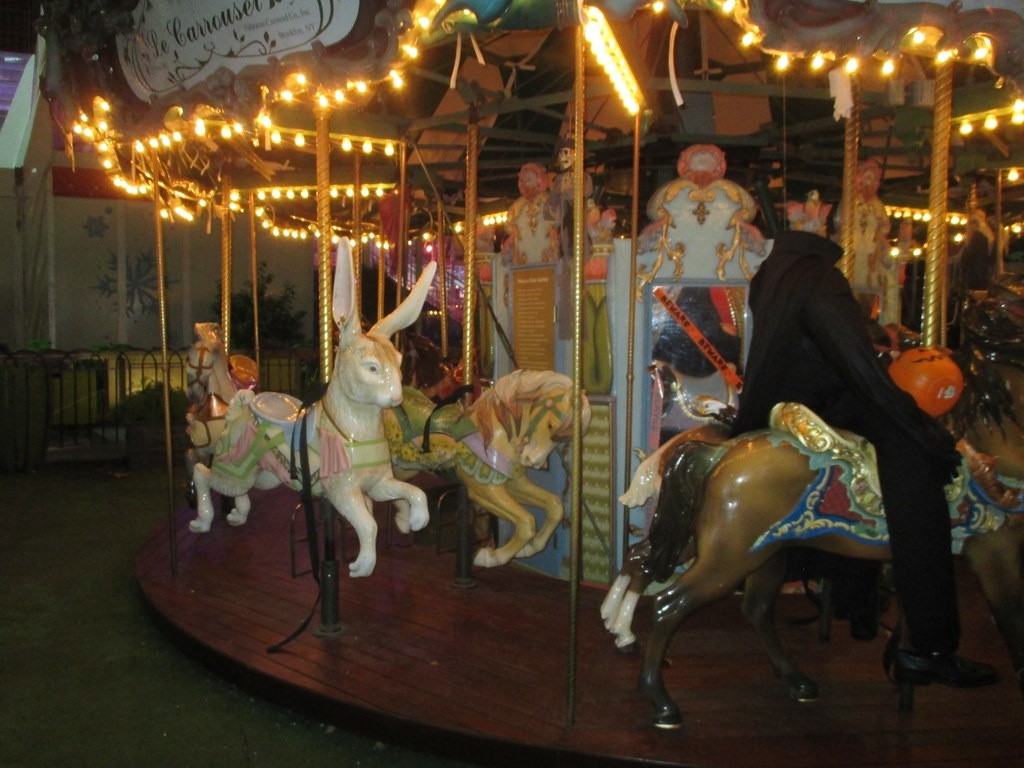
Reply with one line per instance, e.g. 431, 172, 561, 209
748, 230, 1004, 691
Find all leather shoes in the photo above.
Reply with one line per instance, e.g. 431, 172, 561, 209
849, 584, 891, 643
889, 650, 1001, 690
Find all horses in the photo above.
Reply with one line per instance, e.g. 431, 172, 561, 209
600, 272, 1022, 730
186, 323, 261, 514
386, 369, 592, 568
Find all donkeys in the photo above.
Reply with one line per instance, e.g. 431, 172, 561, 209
190, 237, 438, 577
